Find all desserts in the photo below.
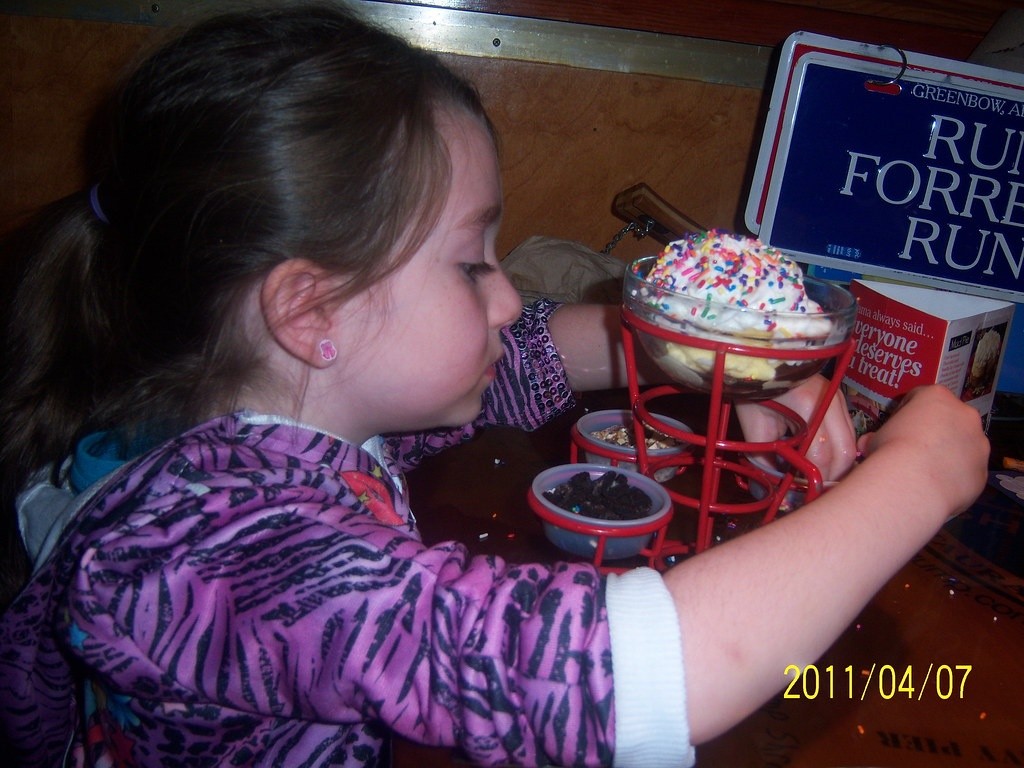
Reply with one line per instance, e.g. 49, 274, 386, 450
622, 226, 836, 400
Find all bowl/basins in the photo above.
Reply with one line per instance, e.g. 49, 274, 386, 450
741, 438, 863, 507
578, 409, 695, 482
531, 463, 672, 560
621, 255, 857, 402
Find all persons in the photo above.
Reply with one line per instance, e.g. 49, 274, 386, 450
0, 11, 989, 768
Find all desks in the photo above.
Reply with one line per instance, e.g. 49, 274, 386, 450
380, 382, 1024, 768
